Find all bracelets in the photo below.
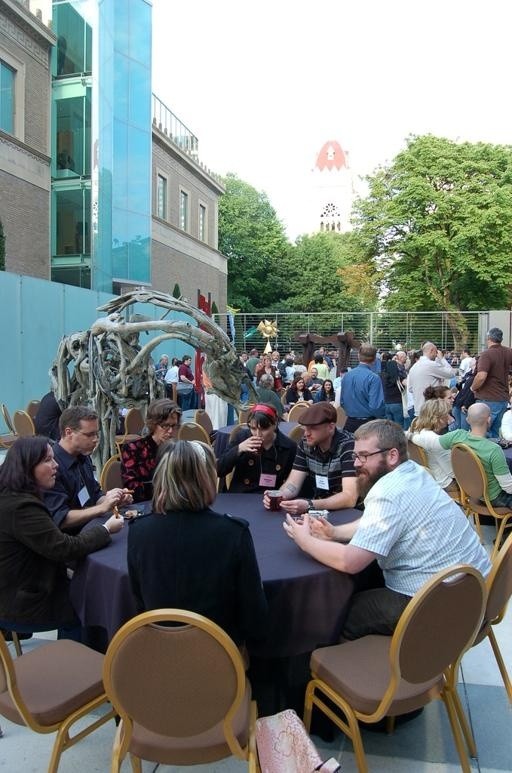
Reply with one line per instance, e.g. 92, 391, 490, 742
303, 497, 315, 512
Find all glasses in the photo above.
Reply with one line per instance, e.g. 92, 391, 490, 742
158, 423, 181, 431
71, 428, 100, 439
352, 448, 388, 463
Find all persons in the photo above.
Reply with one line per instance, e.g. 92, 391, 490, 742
401, 398, 458, 492
120, 398, 183, 502
400, 401, 512, 507
32, 406, 134, 535
0, 435, 125, 639
28, 325, 512, 442
126, 436, 281, 712
279, 418, 495, 641
214, 404, 298, 495
264, 401, 364, 514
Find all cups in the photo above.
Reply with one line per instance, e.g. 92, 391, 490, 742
255, 438, 264, 455
267, 491, 284, 512
308, 509, 328, 539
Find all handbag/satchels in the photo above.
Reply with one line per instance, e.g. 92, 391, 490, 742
176, 381, 193, 398
402, 389, 409, 418
256, 708, 340, 773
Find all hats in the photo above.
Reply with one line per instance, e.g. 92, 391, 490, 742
297, 401, 337, 426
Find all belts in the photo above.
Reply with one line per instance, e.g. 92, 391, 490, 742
348, 417, 369, 421
